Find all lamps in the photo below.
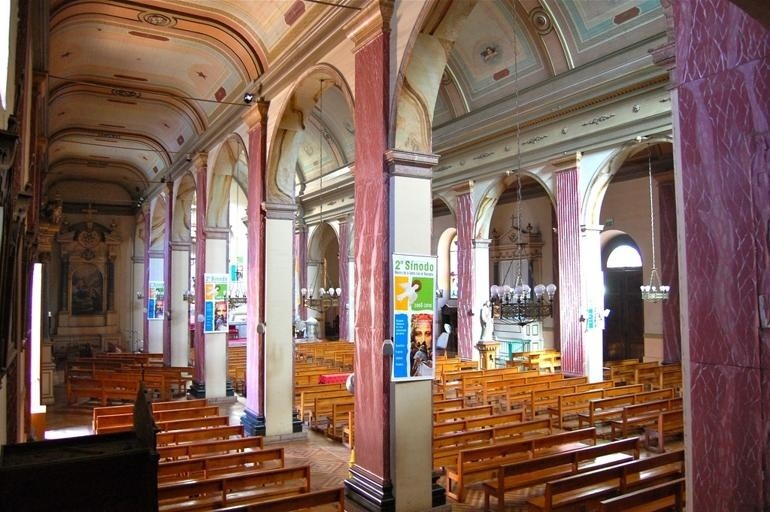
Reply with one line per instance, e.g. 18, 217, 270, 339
301, 80, 342, 314
640, 145, 670, 301
490, 28, 556, 323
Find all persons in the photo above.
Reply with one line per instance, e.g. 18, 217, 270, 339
480, 300, 494, 340
107, 342, 121, 353
207, 285, 220, 298
411, 314, 432, 376
79, 342, 96, 353
397, 279, 423, 304
155, 301, 164, 318
480, 46, 496, 62
72, 277, 101, 311
215, 302, 227, 331
